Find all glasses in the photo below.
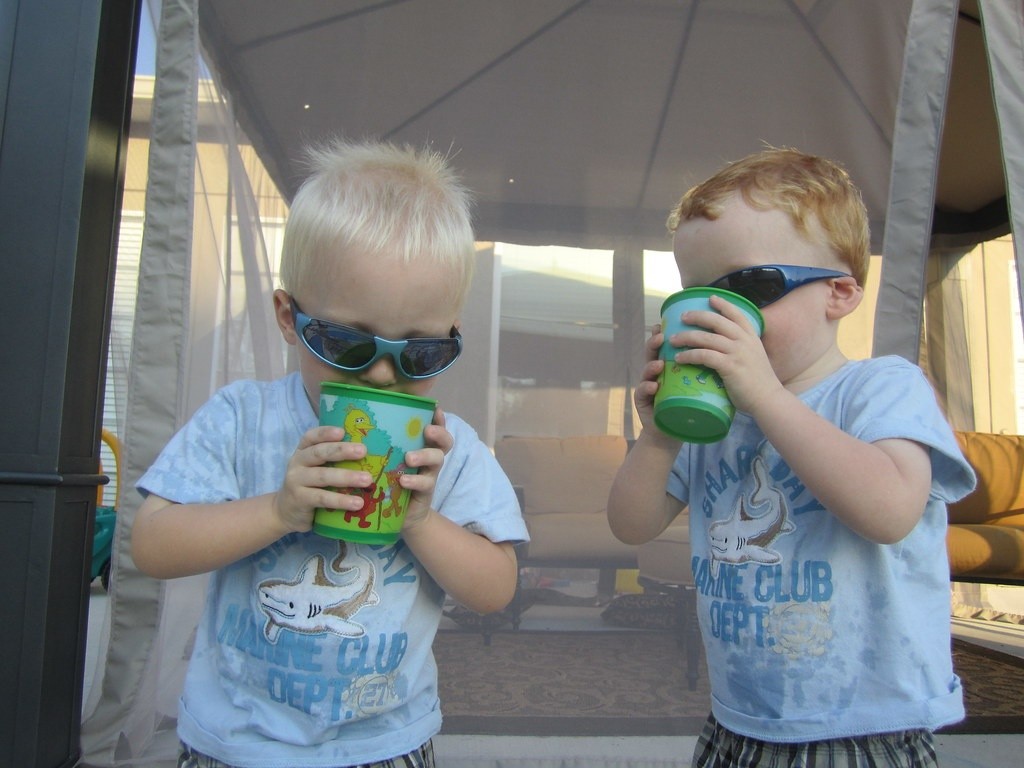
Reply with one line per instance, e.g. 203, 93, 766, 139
704, 264, 857, 309
287, 294, 463, 380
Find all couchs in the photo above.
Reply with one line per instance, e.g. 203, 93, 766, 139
497, 436, 640, 608
948, 432, 1024, 585
635, 526, 699, 688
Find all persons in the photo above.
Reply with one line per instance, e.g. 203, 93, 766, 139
606, 139, 977, 768
127, 137, 532, 768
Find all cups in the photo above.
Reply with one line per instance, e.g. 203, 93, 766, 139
312, 380, 440, 546
652, 285, 765, 442
